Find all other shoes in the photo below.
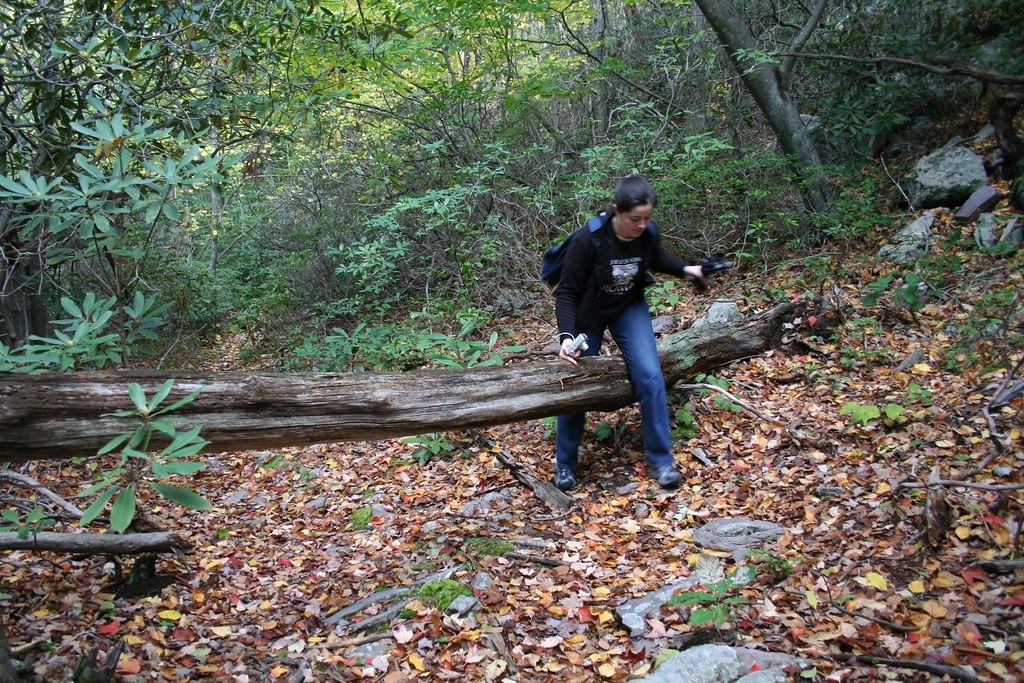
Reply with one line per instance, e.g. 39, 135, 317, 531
557, 467, 575, 491
650, 464, 682, 488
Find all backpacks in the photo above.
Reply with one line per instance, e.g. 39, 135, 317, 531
542, 212, 659, 298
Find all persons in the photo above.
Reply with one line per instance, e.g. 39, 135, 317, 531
555, 175, 707, 492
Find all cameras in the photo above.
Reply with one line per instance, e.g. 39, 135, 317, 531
567, 333, 589, 357
